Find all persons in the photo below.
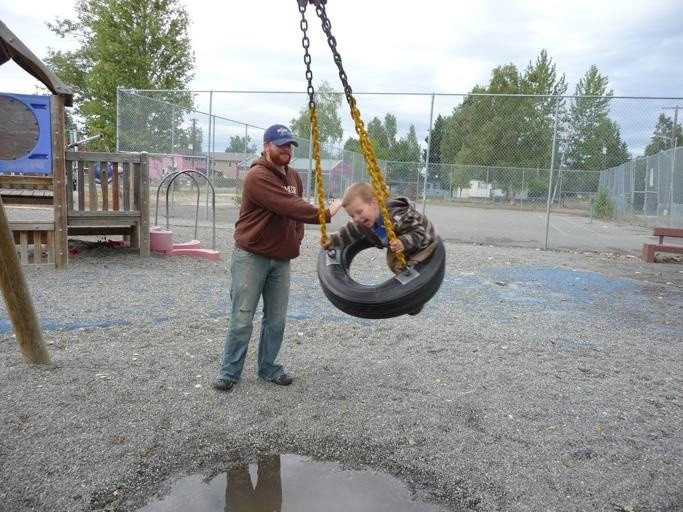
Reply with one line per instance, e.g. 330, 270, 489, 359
320, 181, 435, 316
212, 125, 341, 391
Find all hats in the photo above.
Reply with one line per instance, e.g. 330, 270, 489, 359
264, 125, 298, 147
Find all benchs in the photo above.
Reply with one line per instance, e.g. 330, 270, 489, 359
642, 227, 682, 263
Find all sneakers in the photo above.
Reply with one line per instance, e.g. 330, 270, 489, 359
272, 375, 293, 385
213, 379, 233, 390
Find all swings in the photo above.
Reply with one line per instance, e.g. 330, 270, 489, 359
296, 0, 443, 320
